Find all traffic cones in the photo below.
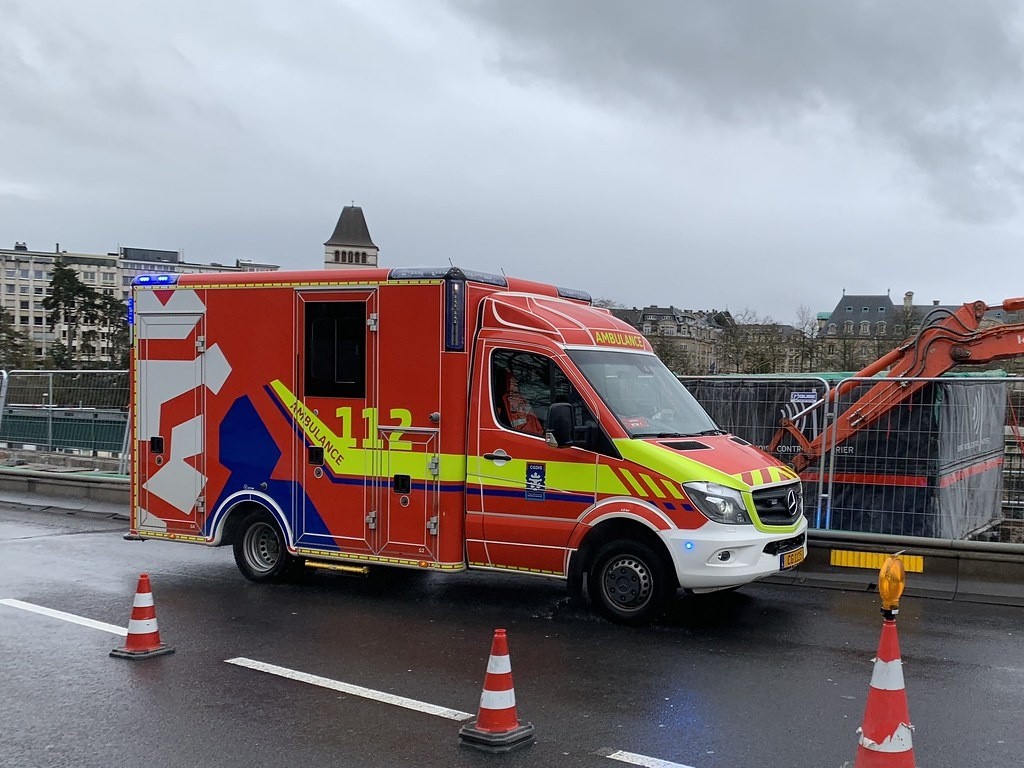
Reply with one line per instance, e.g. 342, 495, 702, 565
853, 556, 915, 768
108, 573, 177, 659
457, 629, 535, 745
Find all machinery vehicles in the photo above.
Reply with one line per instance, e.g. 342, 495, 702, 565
768, 296, 1023, 472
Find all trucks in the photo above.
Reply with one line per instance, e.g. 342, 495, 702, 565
124, 264, 810, 623
